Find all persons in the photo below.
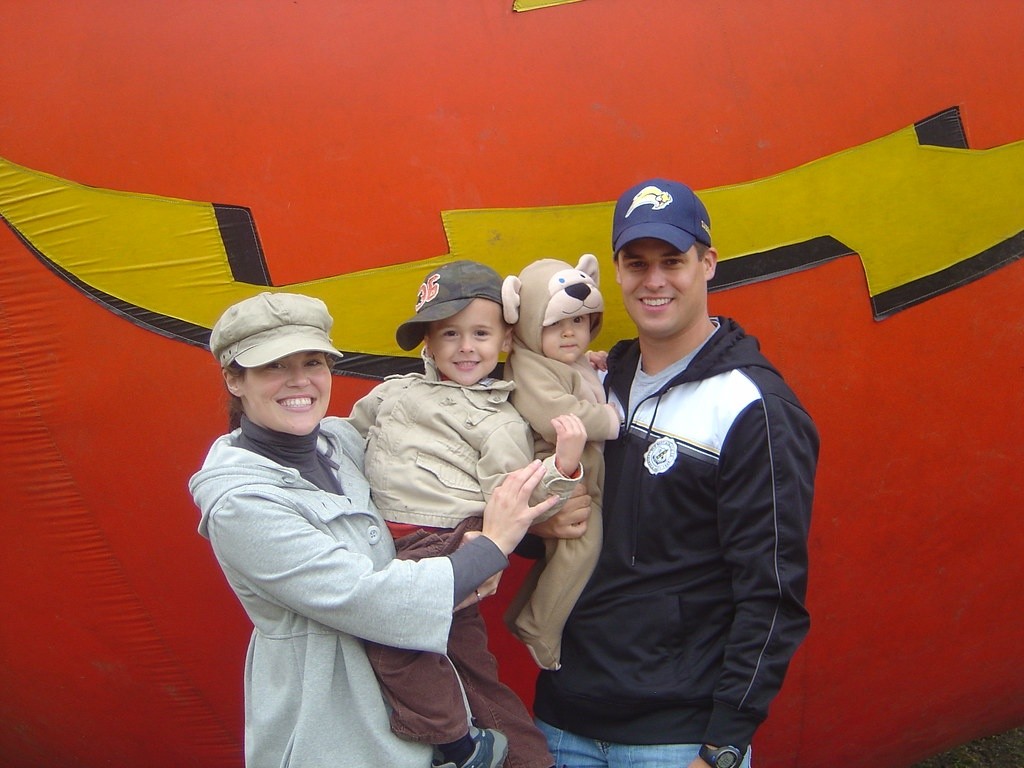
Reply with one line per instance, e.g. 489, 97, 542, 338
515, 176, 820, 768
503, 254, 622, 671
189, 291, 560, 768
341, 261, 588, 768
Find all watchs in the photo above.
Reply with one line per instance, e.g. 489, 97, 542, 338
698, 744, 744, 768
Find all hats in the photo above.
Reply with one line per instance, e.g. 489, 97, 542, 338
209, 292, 343, 371
396, 261, 504, 351
612, 178, 711, 261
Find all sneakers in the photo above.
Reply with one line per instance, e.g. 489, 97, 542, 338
431, 726, 508, 768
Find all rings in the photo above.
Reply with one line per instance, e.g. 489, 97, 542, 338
474, 590, 482, 601
